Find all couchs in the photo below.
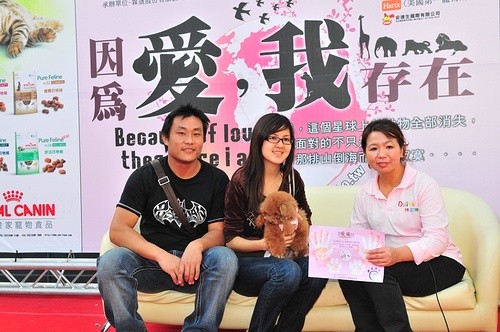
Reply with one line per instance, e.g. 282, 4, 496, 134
100, 186, 500, 332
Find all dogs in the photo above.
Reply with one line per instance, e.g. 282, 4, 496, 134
256, 192, 310, 259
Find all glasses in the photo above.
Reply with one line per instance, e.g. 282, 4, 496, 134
264, 136, 294, 145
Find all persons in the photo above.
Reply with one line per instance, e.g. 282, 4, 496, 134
222, 113, 329, 332
338, 118, 467, 332
97, 102, 239, 332
